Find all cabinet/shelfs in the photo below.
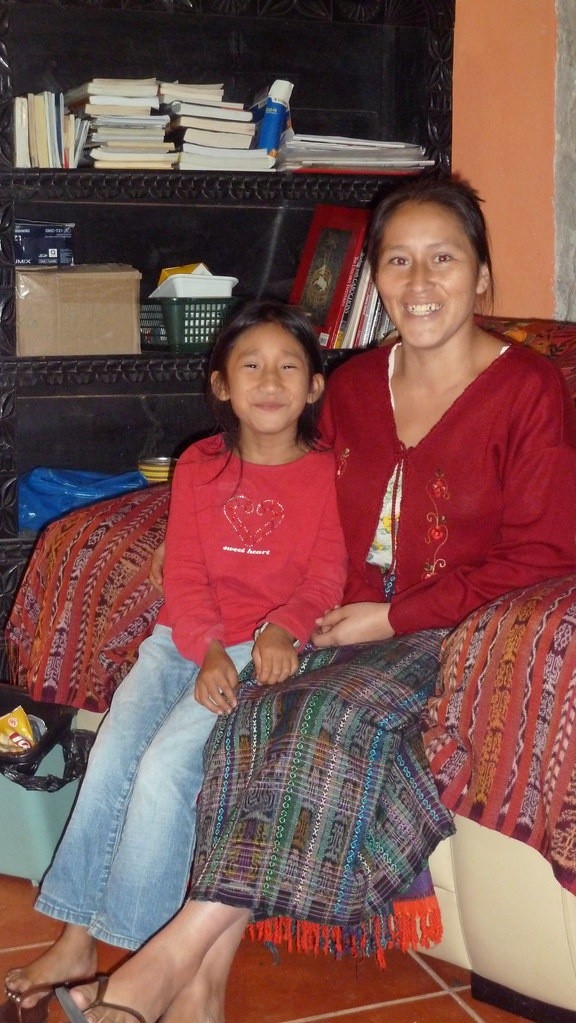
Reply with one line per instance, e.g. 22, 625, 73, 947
2, 0, 457, 642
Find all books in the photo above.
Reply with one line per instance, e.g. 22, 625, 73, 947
0, 73, 428, 349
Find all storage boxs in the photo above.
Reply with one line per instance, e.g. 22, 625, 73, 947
8, 260, 145, 358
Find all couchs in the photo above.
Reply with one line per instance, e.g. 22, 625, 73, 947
0, 315, 575, 1018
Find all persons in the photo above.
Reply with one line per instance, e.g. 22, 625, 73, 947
3, 300, 349, 1023
33, 164, 576, 1023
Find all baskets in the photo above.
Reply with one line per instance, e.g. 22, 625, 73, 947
140, 295, 236, 352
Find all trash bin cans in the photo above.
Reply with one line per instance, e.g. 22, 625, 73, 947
1, 692, 96, 888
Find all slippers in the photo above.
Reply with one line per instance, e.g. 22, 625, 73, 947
0, 972, 56, 1022
55, 971, 147, 1023
